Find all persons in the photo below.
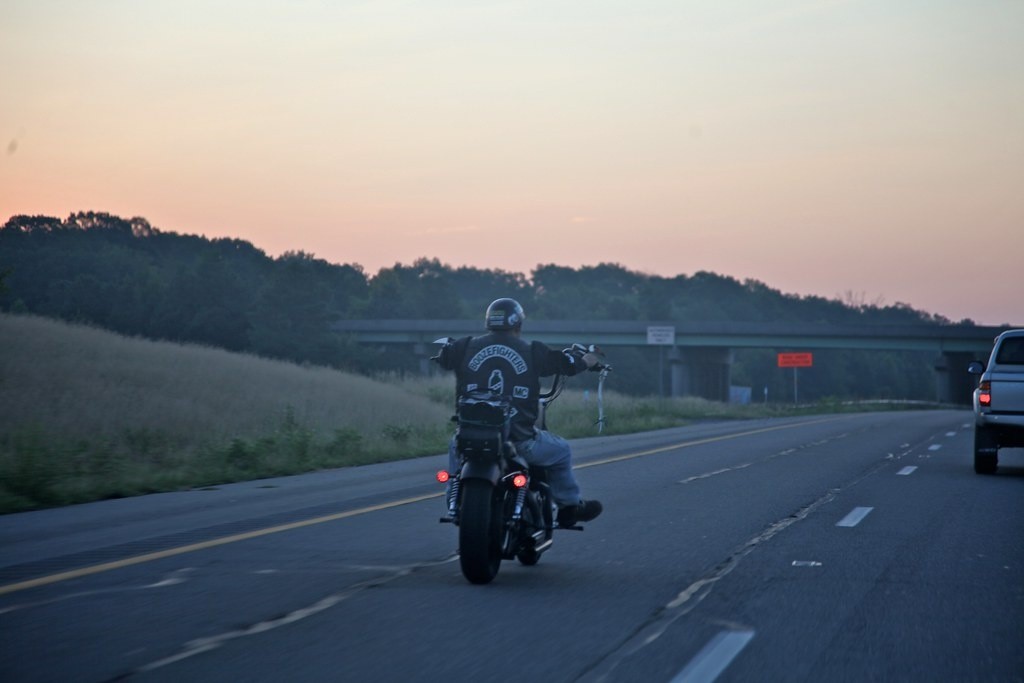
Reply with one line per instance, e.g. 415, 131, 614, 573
436, 298, 600, 527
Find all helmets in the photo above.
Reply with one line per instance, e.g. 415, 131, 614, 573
485, 298, 525, 331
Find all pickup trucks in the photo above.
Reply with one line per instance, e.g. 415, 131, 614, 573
967, 329, 1024, 476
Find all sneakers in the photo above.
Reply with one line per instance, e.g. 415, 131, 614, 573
557, 500, 602, 527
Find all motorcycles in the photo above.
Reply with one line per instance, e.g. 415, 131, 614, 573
429, 337, 613, 585
